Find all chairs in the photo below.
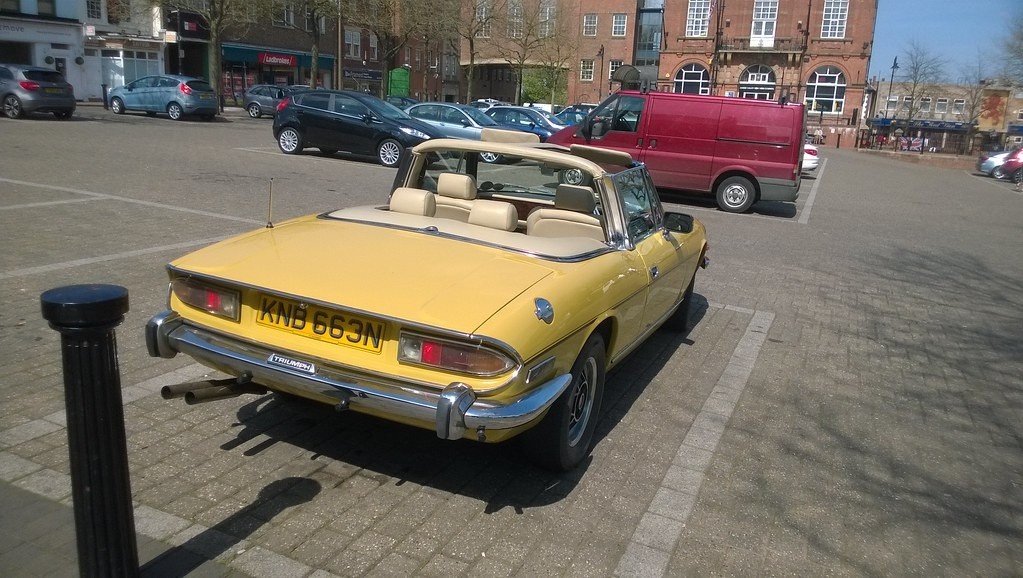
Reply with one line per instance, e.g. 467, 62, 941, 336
390, 171, 609, 244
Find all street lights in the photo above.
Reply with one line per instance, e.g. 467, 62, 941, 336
595, 45, 605, 103
879, 56, 901, 151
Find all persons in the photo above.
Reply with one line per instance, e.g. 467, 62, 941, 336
813, 127, 822, 144
805, 127, 809, 143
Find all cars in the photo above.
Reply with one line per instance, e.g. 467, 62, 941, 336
0, 62, 76, 121
393, 102, 525, 164
272, 89, 449, 169
1001, 147, 1023, 184
975, 150, 1012, 179
147, 128, 709, 475
481, 103, 641, 149
386, 96, 419, 110
242, 85, 291, 119
106, 75, 220, 122
803, 145, 819, 171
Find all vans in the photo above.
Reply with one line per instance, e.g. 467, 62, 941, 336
537, 88, 807, 214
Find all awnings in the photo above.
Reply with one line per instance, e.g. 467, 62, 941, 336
220, 42, 335, 71
